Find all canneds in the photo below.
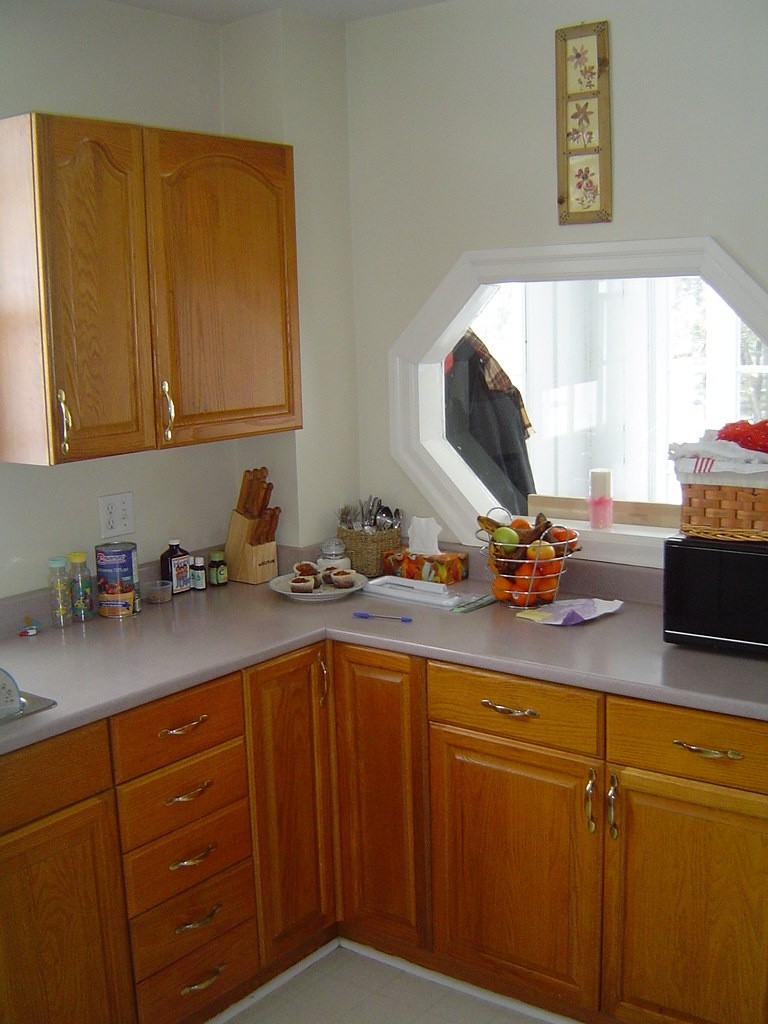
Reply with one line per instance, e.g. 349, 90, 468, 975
94, 541, 143, 619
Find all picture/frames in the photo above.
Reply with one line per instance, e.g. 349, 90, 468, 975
553, 17, 614, 227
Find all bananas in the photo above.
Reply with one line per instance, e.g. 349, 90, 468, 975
477, 512, 582, 572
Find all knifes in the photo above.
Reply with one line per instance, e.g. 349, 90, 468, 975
235, 467, 281, 546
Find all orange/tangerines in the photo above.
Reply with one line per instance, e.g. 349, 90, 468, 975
489, 518, 577, 604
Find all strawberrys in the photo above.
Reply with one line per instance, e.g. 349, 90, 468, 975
97, 576, 134, 594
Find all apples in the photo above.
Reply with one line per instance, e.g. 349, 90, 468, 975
493, 527, 519, 552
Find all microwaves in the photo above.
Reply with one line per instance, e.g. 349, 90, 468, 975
663, 531, 768, 661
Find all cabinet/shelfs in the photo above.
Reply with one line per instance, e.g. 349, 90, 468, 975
0, 720, 137, 1024
0, 111, 306, 467
328, 640, 434, 969
424, 659, 768, 1024
242, 639, 339, 984
107, 671, 260, 1024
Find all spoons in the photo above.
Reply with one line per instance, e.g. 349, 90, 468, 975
393, 508, 402, 528
363, 495, 374, 533
376, 507, 393, 531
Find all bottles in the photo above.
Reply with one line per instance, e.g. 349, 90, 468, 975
190, 555, 196, 588
193, 557, 207, 591
67, 553, 95, 622
208, 551, 228, 586
318, 537, 351, 570
160, 539, 190, 596
48, 556, 72, 628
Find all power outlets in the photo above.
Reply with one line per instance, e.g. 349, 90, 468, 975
96, 491, 136, 542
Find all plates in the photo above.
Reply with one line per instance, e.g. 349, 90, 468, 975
269, 573, 368, 602
0, 669, 20, 718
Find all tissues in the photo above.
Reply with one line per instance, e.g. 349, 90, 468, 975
383, 515, 470, 585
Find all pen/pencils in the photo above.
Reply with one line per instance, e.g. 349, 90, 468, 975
353, 612, 413, 623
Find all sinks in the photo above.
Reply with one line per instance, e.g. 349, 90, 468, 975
0, 689, 57, 724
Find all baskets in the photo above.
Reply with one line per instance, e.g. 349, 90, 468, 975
673, 459, 768, 541
336, 526, 403, 577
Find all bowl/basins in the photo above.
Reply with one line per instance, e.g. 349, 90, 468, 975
319, 566, 341, 584
289, 577, 315, 593
293, 561, 319, 575
143, 581, 172, 603
331, 569, 356, 589
297, 571, 322, 588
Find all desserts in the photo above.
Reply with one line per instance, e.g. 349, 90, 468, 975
289, 561, 355, 593
